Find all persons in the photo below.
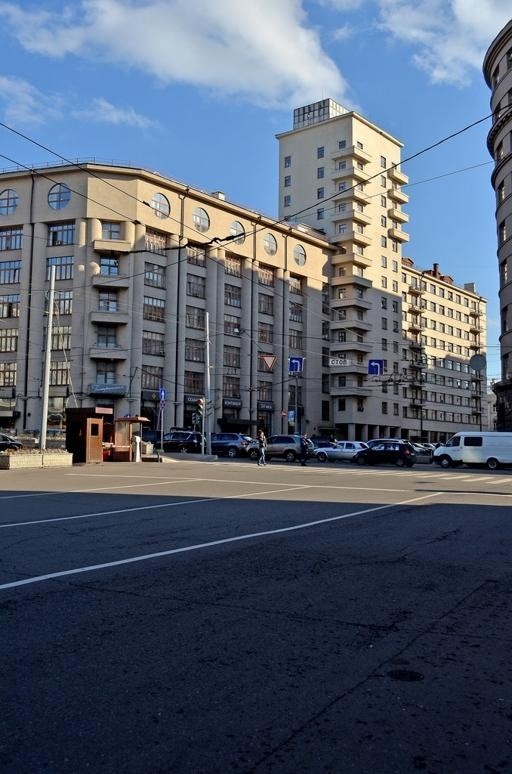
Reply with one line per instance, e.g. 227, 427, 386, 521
257, 431, 268, 466
299, 432, 311, 466
329, 434, 337, 447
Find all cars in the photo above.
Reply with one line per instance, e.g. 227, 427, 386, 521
0, 428, 66, 452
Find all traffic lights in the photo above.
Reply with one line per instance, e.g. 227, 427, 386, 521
196, 399, 204, 417
204, 398, 212, 417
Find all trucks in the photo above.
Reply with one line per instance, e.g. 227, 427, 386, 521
433, 430, 512, 470
